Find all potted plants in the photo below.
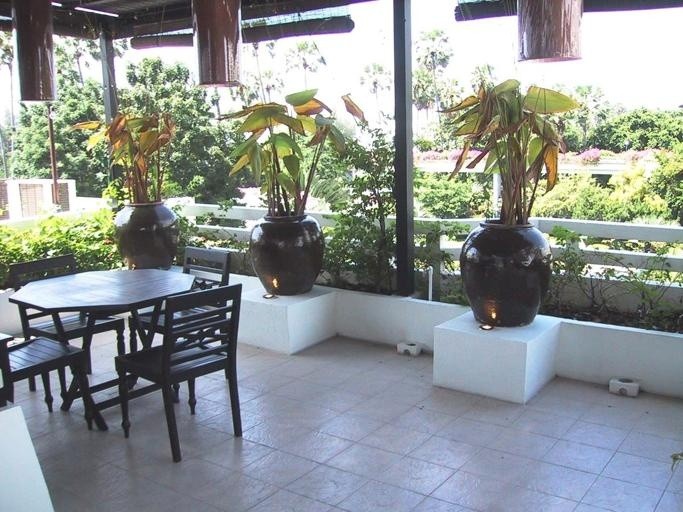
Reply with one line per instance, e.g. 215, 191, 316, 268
437, 65, 581, 328
65, 108, 182, 270
219, 90, 367, 295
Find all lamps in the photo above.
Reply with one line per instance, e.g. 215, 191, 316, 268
190, 0, 242, 87
13, 0, 56, 102
517, 0, 581, 63
